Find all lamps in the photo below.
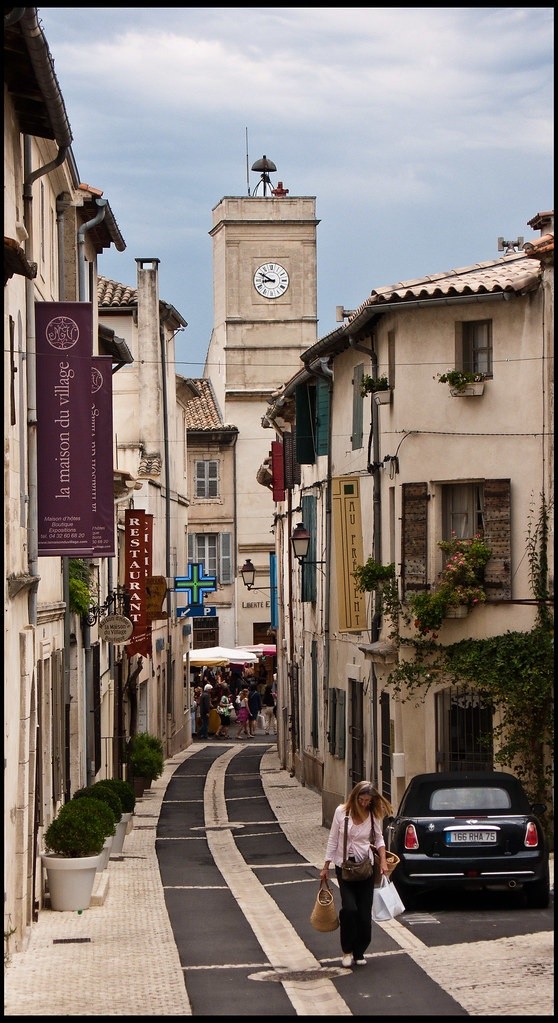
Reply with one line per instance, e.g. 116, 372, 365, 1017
290, 523, 325, 574
241, 559, 277, 591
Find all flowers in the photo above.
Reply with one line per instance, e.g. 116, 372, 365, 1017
436, 583, 486, 606
433, 367, 486, 391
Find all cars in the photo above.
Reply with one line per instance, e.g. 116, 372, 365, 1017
384, 770, 551, 908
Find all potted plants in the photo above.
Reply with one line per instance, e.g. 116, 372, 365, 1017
359, 374, 391, 405
41, 732, 164, 910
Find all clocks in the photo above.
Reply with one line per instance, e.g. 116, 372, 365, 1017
253, 261, 290, 299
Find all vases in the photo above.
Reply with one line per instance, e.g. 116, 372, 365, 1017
443, 603, 467, 618
448, 381, 484, 395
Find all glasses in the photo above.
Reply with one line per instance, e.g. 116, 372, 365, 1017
358, 799, 370, 804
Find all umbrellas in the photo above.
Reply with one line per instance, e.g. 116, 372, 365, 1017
183, 645, 257, 667
235, 644, 277, 658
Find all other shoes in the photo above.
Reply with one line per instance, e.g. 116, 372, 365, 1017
205, 736, 212, 740
236, 736, 242, 739
247, 734, 254, 738
342, 953, 353, 966
355, 959, 367, 965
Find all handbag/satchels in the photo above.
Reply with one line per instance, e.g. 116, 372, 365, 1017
371, 874, 406, 921
217, 706, 227, 714
369, 843, 400, 883
225, 707, 231, 717
311, 876, 339, 932
342, 859, 373, 882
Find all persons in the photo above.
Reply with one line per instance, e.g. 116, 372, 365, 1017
321, 781, 393, 967
193, 663, 279, 740
